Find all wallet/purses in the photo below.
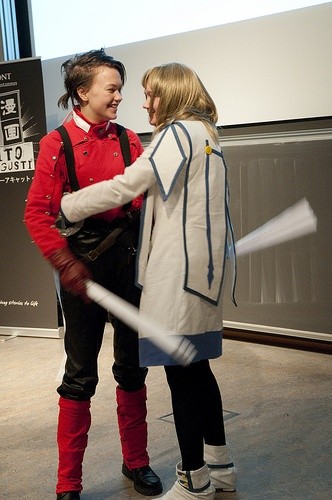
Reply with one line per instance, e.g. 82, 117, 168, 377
78, 236, 111, 273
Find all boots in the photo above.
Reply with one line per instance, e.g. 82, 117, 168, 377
201, 440, 238, 493
158, 462, 217, 500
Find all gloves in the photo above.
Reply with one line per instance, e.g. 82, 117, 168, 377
46, 248, 93, 306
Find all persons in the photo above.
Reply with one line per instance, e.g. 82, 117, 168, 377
56, 61, 238, 500
24, 48, 164, 500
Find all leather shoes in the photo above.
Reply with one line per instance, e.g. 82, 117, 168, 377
56, 493, 80, 500
120, 461, 163, 498
